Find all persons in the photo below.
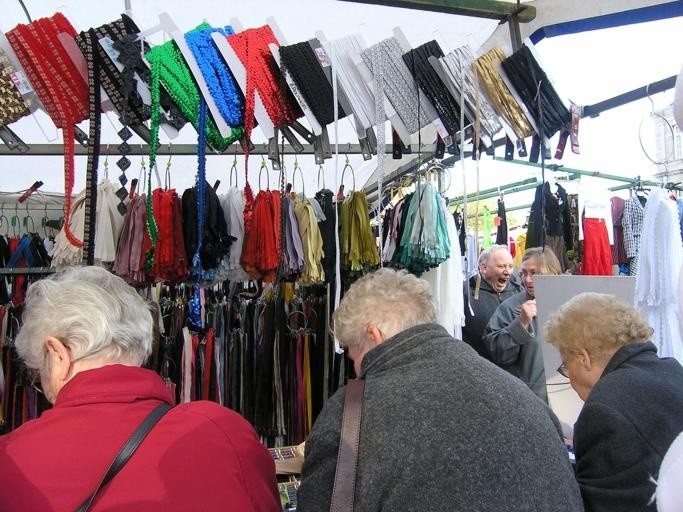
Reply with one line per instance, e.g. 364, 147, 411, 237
1, 264, 283, 512
481, 245, 571, 404
295, 262, 589, 512
460, 244, 526, 359
543, 292, 683, 512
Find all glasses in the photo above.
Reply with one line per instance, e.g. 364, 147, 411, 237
557, 361, 570, 379
518, 271, 543, 277
31, 370, 43, 394
